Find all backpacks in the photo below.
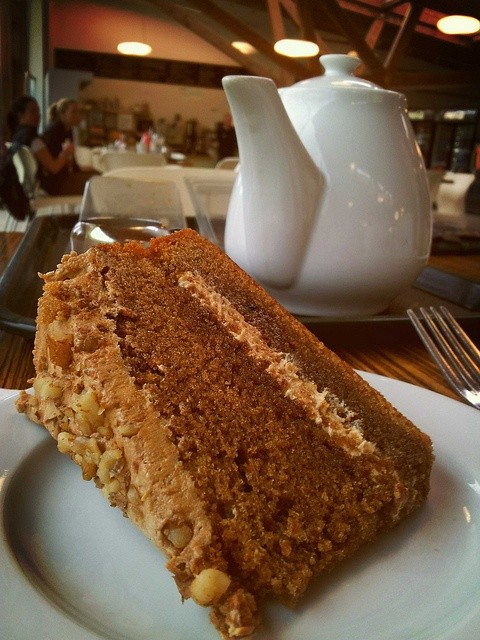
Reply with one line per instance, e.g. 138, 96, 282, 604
0, 141, 32, 221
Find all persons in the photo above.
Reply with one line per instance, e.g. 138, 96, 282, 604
6, 96, 101, 196
42, 97, 101, 176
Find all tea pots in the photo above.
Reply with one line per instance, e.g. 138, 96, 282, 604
222, 53, 432, 317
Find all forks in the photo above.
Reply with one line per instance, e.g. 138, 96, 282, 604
407, 307, 480, 408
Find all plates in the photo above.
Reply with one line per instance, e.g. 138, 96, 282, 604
0, 369, 480, 639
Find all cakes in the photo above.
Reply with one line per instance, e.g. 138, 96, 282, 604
12, 229, 433, 640
